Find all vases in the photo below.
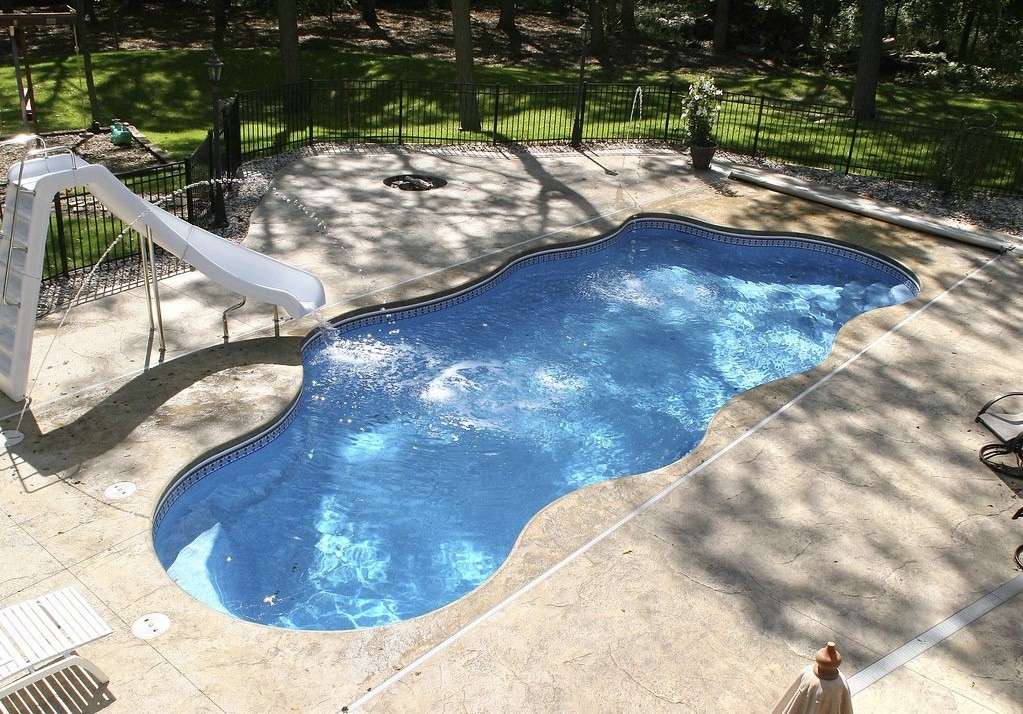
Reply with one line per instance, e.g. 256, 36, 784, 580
688, 141, 718, 169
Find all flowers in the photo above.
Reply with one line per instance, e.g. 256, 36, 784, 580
677, 76, 724, 147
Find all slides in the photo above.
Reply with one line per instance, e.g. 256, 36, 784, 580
77, 153, 327, 321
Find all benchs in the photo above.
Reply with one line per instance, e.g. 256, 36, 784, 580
0, 584, 114, 701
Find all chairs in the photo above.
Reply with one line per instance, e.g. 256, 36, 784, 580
974, 391, 1023, 478
1011, 507, 1023, 569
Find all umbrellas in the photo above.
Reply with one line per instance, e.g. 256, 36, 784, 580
771, 642, 853, 714
